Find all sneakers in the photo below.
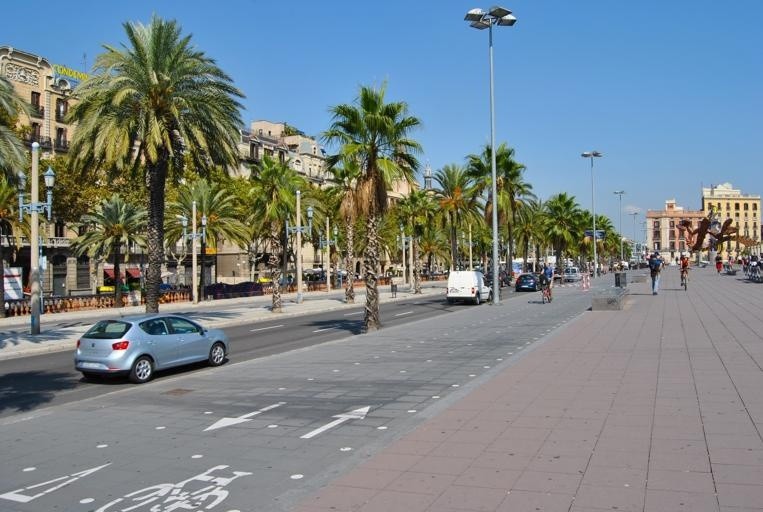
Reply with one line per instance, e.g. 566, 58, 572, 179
653, 292, 658, 295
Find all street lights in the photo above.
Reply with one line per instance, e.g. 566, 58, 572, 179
582, 150, 603, 274
182, 201, 206, 304
396, 223, 412, 284
16, 142, 56, 337
614, 190, 626, 236
465, 6, 516, 306
285, 190, 313, 305
319, 216, 338, 292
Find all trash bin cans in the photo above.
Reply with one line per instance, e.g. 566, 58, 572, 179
391, 284, 397, 298
615, 273, 627, 289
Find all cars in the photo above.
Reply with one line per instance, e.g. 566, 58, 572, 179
74, 314, 229, 383
515, 272, 543, 293
485, 270, 511, 288
589, 259, 649, 274
553, 266, 584, 284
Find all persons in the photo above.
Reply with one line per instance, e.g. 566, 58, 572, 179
390, 275, 397, 298
715, 252, 758, 275
425, 270, 429, 281
611, 261, 624, 273
679, 255, 690, 285
649, 252, 665, 295
500, 250, 553, 304
419, 270, 423, 281
287, 272, 294, 292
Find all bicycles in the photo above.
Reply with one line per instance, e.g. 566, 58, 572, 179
680, 267, 689, 292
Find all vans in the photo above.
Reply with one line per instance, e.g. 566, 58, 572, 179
446, 270, 493, 306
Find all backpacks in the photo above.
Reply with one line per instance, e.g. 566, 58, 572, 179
681, 259, 688, 268
652, 256, 665, 272
